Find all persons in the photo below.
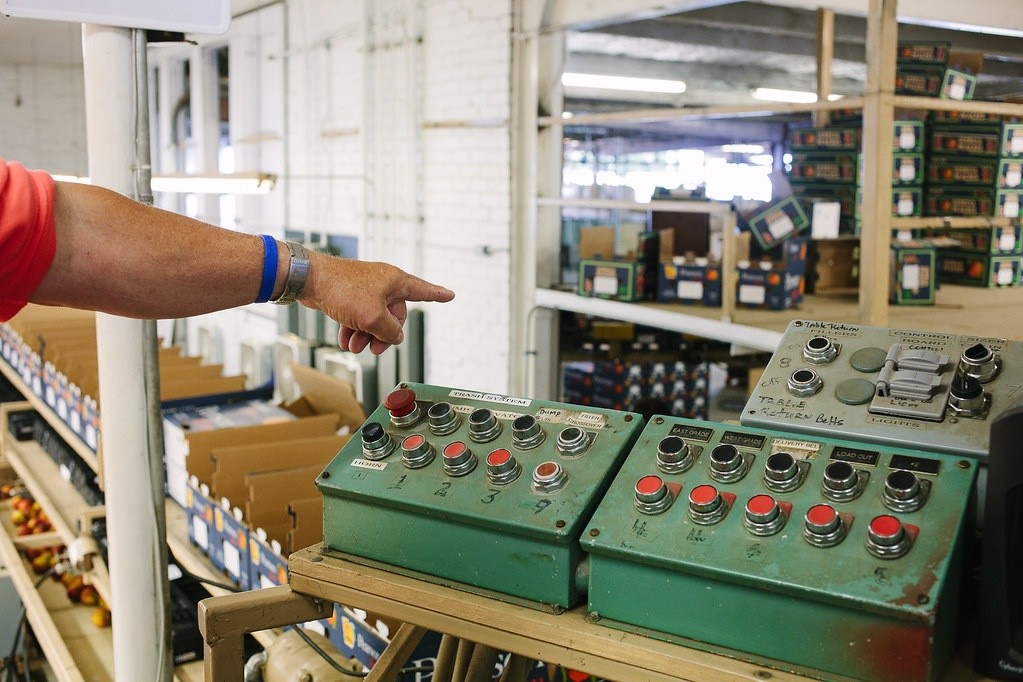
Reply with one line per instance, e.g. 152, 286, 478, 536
0, 151, 455, 355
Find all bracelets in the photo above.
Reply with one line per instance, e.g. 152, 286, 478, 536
268, 241, 309, 305
253, 235, 278, 305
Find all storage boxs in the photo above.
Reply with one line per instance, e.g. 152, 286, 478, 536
0, 296, 436, 676
556, 38, 1023, 426
